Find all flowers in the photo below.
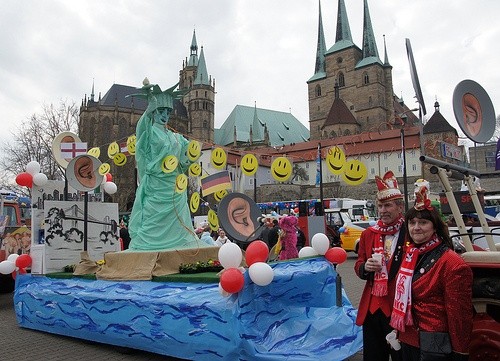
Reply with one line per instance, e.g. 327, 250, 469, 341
179, 259, 220, 269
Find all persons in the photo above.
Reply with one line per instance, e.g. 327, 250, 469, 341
392, 179, 472, 361
0, 231, 31, 255
354, 171, 411, 361
119, 215, 130, 249
193, 215, 306, 255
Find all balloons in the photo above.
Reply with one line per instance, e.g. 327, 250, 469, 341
25, 161, 41, 173
31, 173, 48, 185
249, 262, 274, 286
339, 226, 349, 235
325, 247, 348, 264
218, 239, 243, 268
299, 246, 316, 258
220, 267, 244, 293
102, 173, 118, 194
311, 232, 329, 257
15, 172, 33, 188
245, 240, 270, 268
0, 253, 32, 279
294, 208, 299, 213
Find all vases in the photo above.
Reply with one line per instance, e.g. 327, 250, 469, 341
179, 266, 223, 274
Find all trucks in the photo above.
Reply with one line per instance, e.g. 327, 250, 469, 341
328, 197, 379, 222
324, 207, 367, 253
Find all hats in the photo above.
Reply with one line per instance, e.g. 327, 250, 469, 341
375, 171, 404, 200
